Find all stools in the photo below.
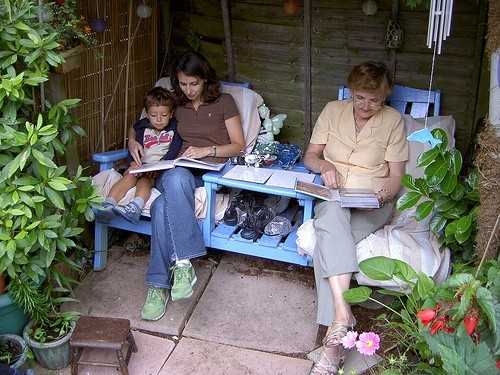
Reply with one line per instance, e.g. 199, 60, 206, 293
69, 316, 138, 375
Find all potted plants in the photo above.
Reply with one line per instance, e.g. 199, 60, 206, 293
0, 0, 106, 375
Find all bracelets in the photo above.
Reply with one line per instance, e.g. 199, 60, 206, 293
377, 189, 391, 204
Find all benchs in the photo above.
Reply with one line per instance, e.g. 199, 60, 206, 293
91, 79, 440, 272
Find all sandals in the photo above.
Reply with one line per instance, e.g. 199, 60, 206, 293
309, 342, 344, 375
113, 202, 141, 224
92, 201, 115, 224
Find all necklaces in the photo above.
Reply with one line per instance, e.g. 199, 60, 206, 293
355, 118, 367, 133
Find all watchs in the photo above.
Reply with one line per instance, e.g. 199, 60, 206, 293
210, 144, 217, 158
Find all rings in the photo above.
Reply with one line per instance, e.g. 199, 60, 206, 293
187, 152, 192, 156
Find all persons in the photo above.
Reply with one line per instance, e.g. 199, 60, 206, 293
257, 104, 287, 145
98, 86, 183, 227
128, 52, 246, 321
303, 59, 408, 375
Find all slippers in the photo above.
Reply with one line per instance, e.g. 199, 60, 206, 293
323, 318, 358, 347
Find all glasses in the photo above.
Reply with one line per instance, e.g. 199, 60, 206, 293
352, 92, 384, 111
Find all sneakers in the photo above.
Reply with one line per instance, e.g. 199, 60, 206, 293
169, 264, 198, 301
141, 287, 168, 321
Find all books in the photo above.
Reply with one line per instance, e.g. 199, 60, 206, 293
129, 156, 228, 174
294, 177, 379, 211
